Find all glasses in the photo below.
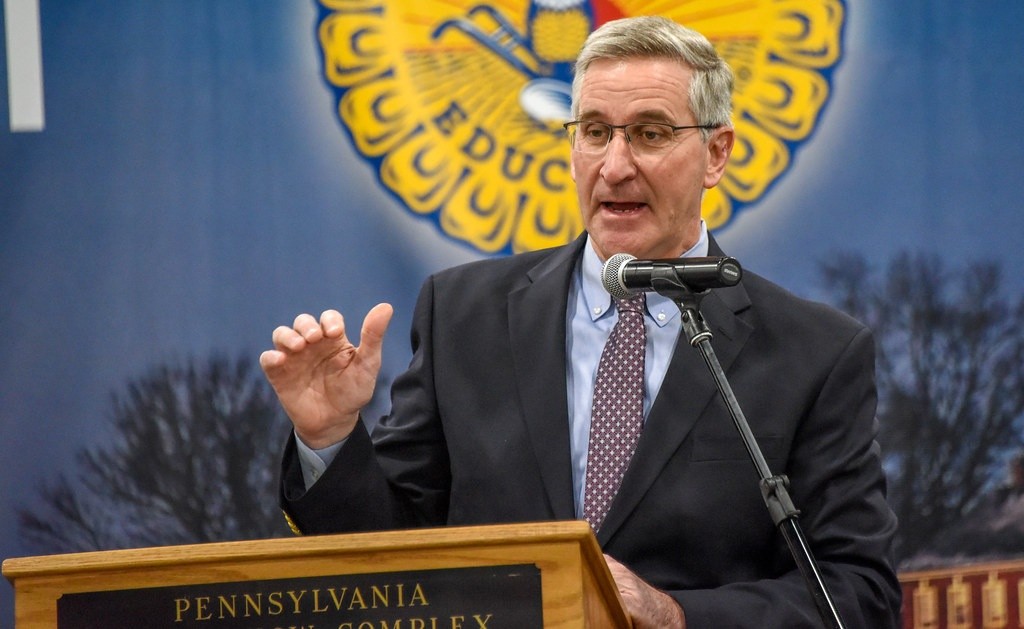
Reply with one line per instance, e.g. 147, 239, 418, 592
564, 120, 730, 151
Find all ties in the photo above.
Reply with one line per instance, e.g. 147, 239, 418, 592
583, 290, 646, 533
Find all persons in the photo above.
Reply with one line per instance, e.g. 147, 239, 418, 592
253, 15, 906, 629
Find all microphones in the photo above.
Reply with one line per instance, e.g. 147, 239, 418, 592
601, 252, 742, 299
